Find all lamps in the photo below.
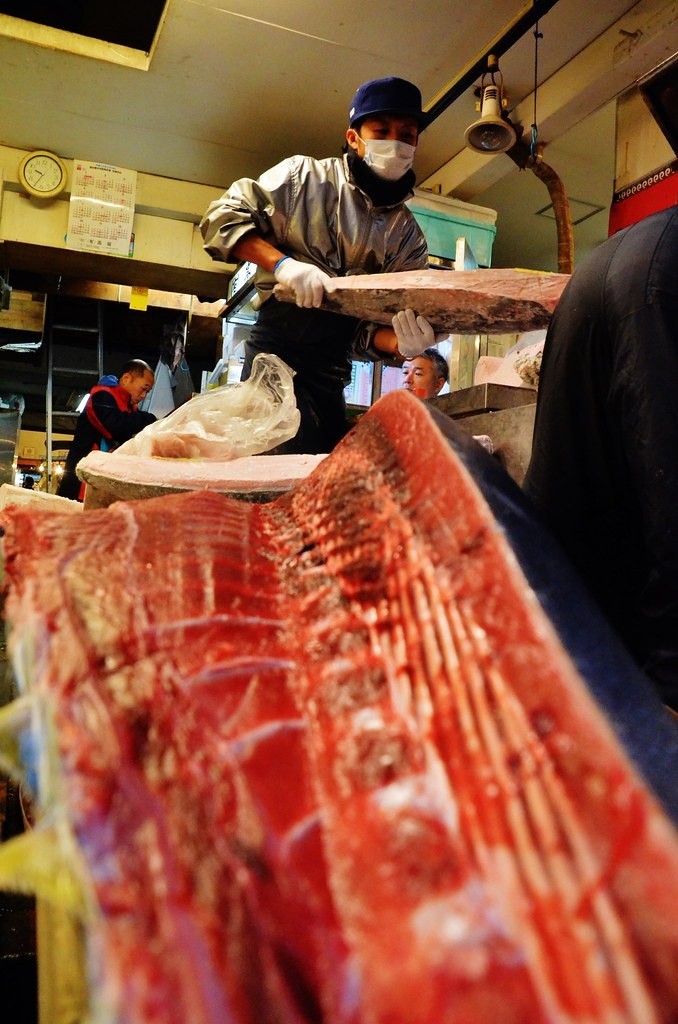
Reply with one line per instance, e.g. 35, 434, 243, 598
464, 53, 517, 155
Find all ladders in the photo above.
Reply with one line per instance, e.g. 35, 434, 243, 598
45, 297, 106, 497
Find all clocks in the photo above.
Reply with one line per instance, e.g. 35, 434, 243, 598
18, 150, 67, 199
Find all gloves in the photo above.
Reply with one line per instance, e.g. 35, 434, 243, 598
274, 256, 335, 308
391, 308, 450, 360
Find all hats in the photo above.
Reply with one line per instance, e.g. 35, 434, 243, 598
348, 76, 428, 128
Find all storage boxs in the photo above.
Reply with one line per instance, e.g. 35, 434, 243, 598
404, 188, 498, 225
407, 205, 497, 269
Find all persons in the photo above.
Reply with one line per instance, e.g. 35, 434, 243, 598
55, 359, 158, 502
400, 349, 449, 399
200, 77, 451, 456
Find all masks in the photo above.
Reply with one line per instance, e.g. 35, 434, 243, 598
351, 129, 415, 180
